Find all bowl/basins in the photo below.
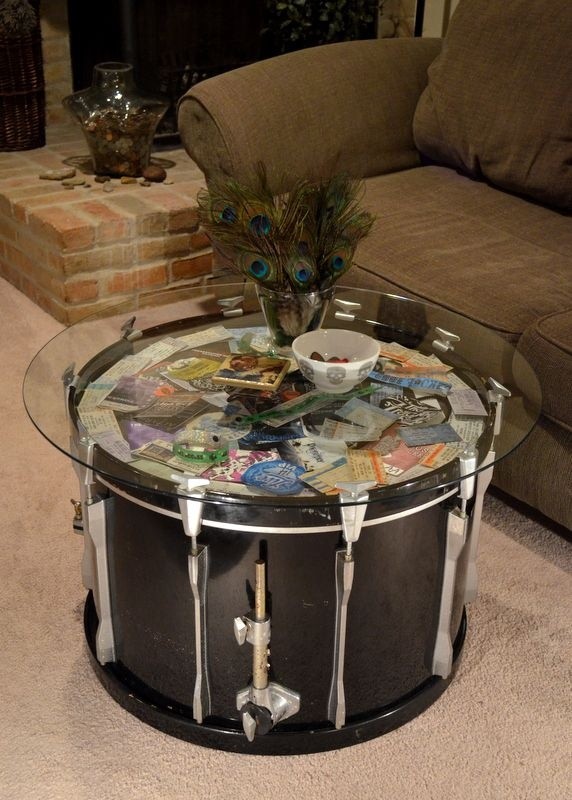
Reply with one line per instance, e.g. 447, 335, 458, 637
291, 329, 381, 394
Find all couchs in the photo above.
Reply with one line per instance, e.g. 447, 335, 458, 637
178, 0, 571, 542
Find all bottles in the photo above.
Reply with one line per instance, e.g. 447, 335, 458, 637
62, 61, 171, 178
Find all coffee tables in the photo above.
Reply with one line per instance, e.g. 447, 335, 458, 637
21, 283, 544, 758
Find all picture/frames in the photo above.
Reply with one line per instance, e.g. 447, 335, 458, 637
213, 352, 290, 391
